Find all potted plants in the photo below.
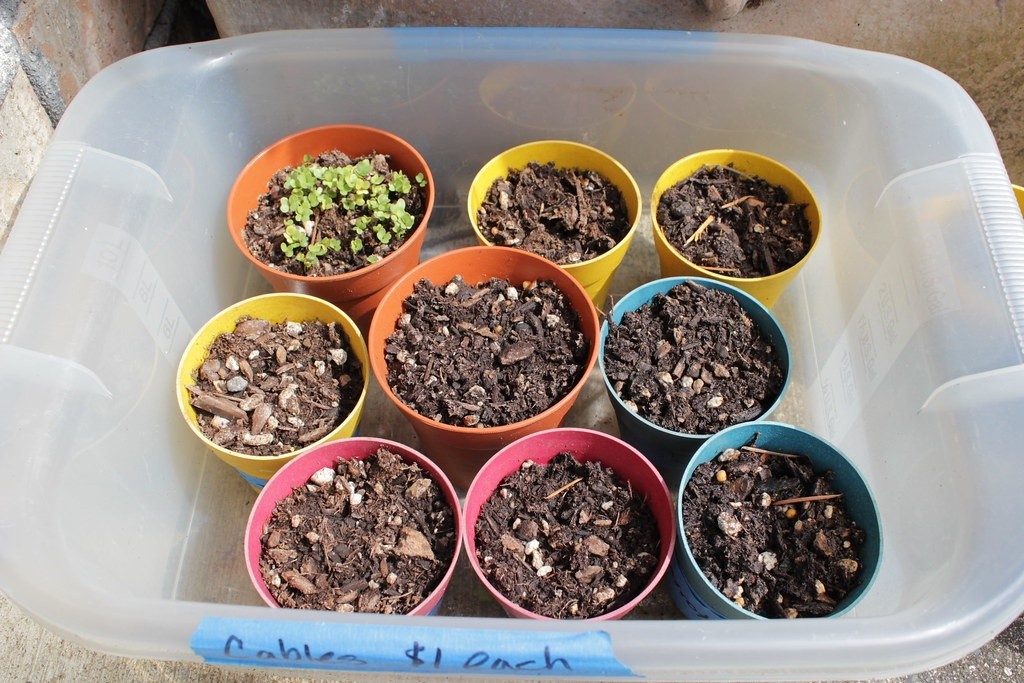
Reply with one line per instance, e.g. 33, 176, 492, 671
176, 125, 883, 620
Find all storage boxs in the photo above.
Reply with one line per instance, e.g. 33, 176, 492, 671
0, 28, 1024, 683
